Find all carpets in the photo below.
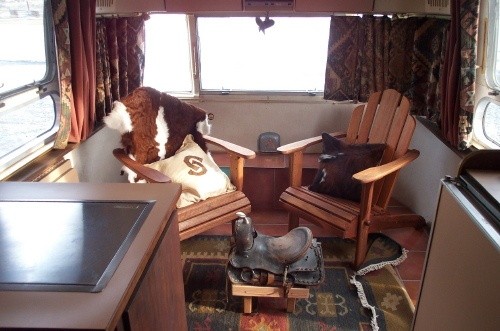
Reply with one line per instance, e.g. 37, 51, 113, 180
180, 233, 416, 331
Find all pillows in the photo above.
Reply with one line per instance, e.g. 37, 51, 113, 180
308, 133, 388, 204
142, 134, 237, 208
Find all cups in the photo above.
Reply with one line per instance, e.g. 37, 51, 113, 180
308, 87, 316, 96
221, 84, 230, 95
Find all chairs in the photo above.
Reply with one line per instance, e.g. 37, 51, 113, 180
276, 89, 427, 270
103, 87, 257, 242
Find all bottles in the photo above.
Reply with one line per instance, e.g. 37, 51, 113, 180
457, 115, 472, 151
